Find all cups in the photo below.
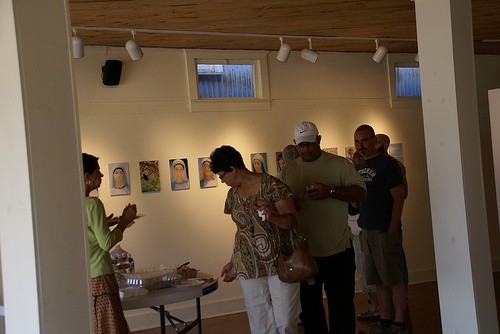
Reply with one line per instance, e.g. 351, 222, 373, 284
304, 181, 319, 198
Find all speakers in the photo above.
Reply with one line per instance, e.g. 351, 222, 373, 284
101, 59, 122, 85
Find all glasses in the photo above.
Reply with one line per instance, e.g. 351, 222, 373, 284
219, 169, 227, 182
296, 140, 317, 148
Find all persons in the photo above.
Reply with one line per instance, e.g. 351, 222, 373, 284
275, 121, 368, 334
347, 124, 412, 334
82, 153, 140, 334
209, 145, 302, 334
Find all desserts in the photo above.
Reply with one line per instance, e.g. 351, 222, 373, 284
177, 264, 197, 279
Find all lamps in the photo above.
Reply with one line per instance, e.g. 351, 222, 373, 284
372, 40, 388, 64
72, 27, 84, 59
275, 37, 291, 63
126, 31, 144, 61
301, 38, 320, 63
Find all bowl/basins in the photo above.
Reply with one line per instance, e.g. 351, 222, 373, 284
176, 268, 198, 278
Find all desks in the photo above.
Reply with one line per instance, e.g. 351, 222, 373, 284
118, 277, 218, 334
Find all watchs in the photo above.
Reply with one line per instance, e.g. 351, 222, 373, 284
330, 184, 335, 197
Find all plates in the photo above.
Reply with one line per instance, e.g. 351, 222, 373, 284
171, 278, 205, 288
107, 213, 142, 223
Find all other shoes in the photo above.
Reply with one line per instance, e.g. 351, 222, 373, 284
357, 308, 382, 322
358, 321, 393, 334
390, 325, 408, 334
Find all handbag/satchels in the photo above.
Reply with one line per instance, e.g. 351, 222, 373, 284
275, 214, 319, 283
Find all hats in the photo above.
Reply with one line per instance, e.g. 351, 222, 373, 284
294, 121, 318, 145
